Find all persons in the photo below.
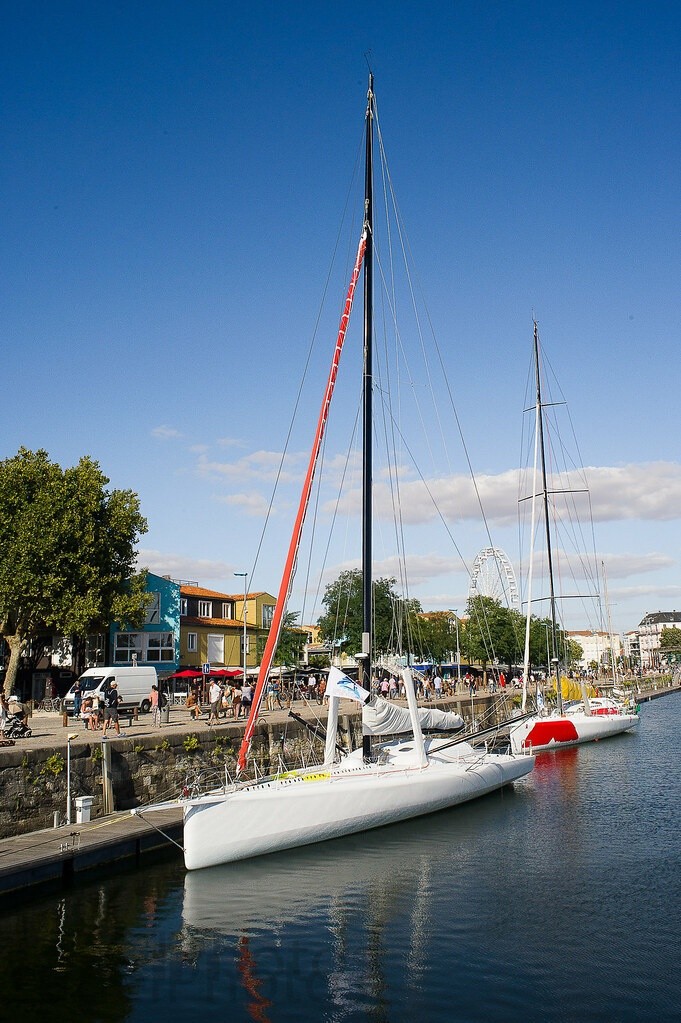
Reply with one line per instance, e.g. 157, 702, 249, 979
148, 685, 158, 726
463, 672, 482, 696
190, 678, 201, 692
0, 687, 9, 733
103, 680, 125, 739
272, 679, 284, 709
308, 674, 327, 700
373, 674, 443, 701
79, 696, 105, 731
499, 672, 506, 687
205, 678, 256, 726
186, 693, 205, 720
512, 670, 547, 688
73, 681, 84, 716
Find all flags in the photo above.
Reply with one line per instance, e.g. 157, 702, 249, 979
325, 666, 369, 704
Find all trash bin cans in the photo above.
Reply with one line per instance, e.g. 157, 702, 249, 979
160, 706, 169, 723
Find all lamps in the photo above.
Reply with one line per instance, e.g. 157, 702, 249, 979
181, 655, 185, 659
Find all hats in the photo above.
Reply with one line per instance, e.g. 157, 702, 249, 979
384, 678, 388, 681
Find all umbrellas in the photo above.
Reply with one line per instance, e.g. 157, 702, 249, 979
167, 670, 244, 693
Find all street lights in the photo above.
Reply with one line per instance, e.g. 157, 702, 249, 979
232, 572, 247, 686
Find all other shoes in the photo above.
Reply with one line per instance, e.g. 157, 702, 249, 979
205, 721, 212, 726
114, 734, 121, 738
103, 735, 108, 739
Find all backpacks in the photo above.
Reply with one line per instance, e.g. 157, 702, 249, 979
104, 689, 116, 708
158, 692, 167, 707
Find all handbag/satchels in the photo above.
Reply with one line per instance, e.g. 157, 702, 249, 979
232, 696, 241, 704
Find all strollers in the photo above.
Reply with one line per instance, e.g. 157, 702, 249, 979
1, 712, 32, 739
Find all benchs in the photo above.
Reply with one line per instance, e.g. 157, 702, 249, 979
191, 705, 240, 720
82, 713, 138, 730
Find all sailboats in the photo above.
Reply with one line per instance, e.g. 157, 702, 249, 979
507, 292, 640, 754
128, 70, 537, 872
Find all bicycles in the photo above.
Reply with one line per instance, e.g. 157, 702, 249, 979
37, 697, 61, 712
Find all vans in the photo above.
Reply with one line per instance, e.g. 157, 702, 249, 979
62, 665, 159, 718
